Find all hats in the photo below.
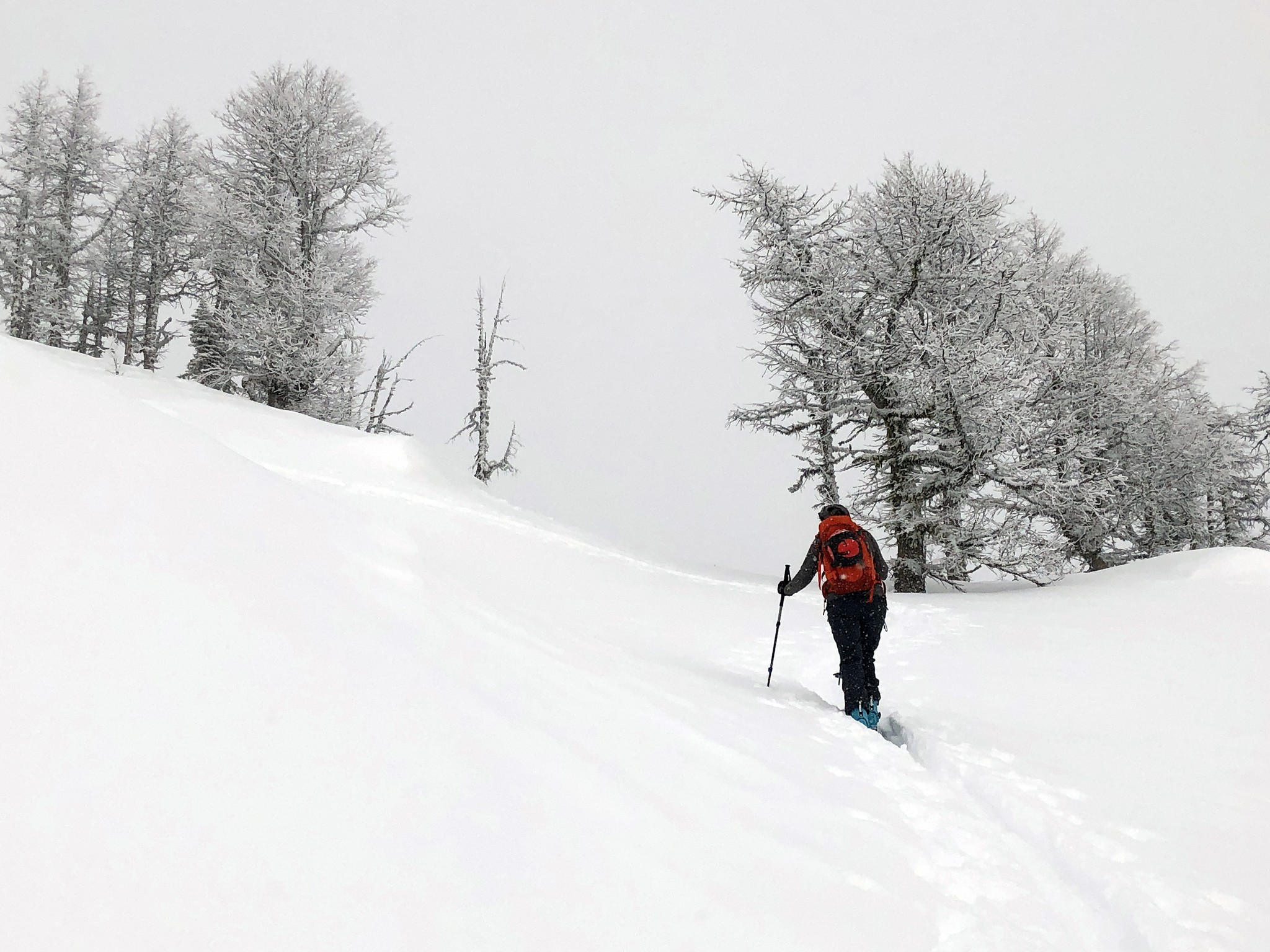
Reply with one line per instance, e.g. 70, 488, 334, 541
821, 504, 850, 522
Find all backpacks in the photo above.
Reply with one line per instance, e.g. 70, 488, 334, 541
819, 515, 883, 595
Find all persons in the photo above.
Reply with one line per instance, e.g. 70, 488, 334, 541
776, 504, 889, 730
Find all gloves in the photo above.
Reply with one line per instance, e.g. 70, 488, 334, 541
777, 580, 790, 596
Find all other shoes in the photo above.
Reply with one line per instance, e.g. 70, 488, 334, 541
866, 701, 880, 730
851, 707, 870, 727
